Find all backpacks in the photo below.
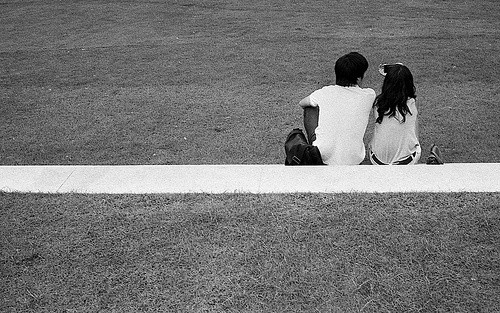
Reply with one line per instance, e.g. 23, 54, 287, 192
284, 128, 323, 165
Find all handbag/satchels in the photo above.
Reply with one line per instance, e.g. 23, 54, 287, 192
427, 144, 444, 164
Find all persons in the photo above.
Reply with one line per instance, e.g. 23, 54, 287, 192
368, 63, 422, 166
298, 51, 375, 167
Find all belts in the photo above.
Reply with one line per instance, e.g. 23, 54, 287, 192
371, 151, 416, 165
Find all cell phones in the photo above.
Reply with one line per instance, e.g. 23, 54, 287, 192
384, 64, 400, 73
